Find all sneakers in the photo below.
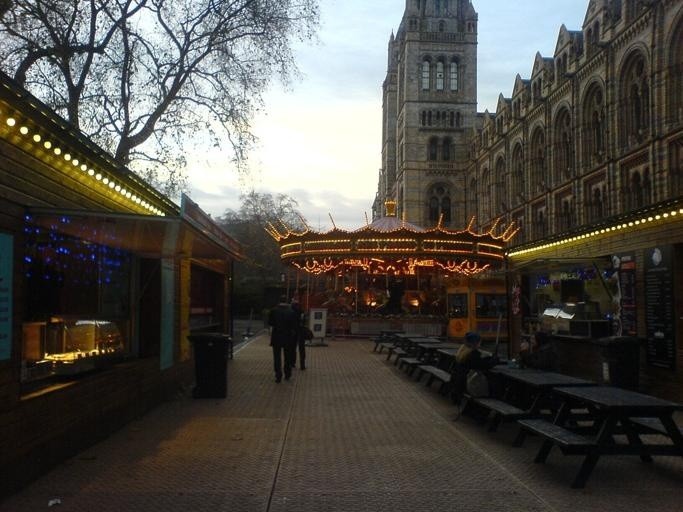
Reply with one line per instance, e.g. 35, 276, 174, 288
275, 364, 307, 383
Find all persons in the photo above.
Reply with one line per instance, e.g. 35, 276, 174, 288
448, 332, 499, 399
519, 331, 560, 373
290, 302, 313, 370
268, 294, 297, 384
480, 296, 504, 313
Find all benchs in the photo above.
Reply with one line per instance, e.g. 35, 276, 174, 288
592, 405, 682, 440
371, 337, 513, 394
474, 395, 526, 419
517, 416, 597, 448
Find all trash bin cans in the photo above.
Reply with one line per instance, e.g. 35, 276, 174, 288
589, 335, 645, 392
187, 333, 232, 399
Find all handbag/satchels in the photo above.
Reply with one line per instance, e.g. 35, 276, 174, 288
466, 368, 490, 399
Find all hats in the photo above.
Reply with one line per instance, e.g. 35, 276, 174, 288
464, 331, 482, 345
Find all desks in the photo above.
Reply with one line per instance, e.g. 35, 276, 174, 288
534, 386, 682, 488
503, 369, 597, 446
